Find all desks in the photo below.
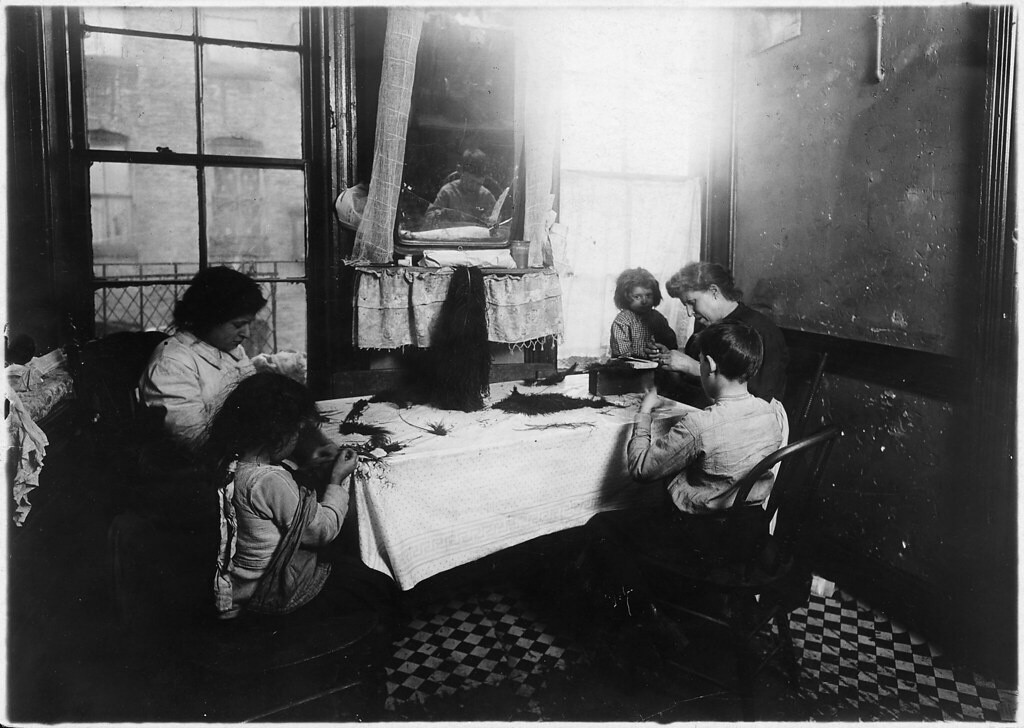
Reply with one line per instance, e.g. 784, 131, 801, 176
305, 369, 704, 639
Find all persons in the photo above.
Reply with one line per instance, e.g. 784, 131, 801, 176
202, 368, 411, 672
139, 264, 277, 462
626, 319, 791, 566
608, 269, 678, 400
643, 257, 794, 413
423, 149, 506, 226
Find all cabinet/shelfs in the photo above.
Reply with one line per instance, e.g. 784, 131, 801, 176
6, 385, 96, 721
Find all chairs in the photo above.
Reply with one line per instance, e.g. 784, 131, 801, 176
586, 345, 846, 722
86, 340, 389, 722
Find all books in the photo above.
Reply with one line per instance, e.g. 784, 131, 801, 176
610, 355, 660, 370
442, 183, 509, 228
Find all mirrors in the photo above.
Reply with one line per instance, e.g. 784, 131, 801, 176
393, 12, 525, 249
